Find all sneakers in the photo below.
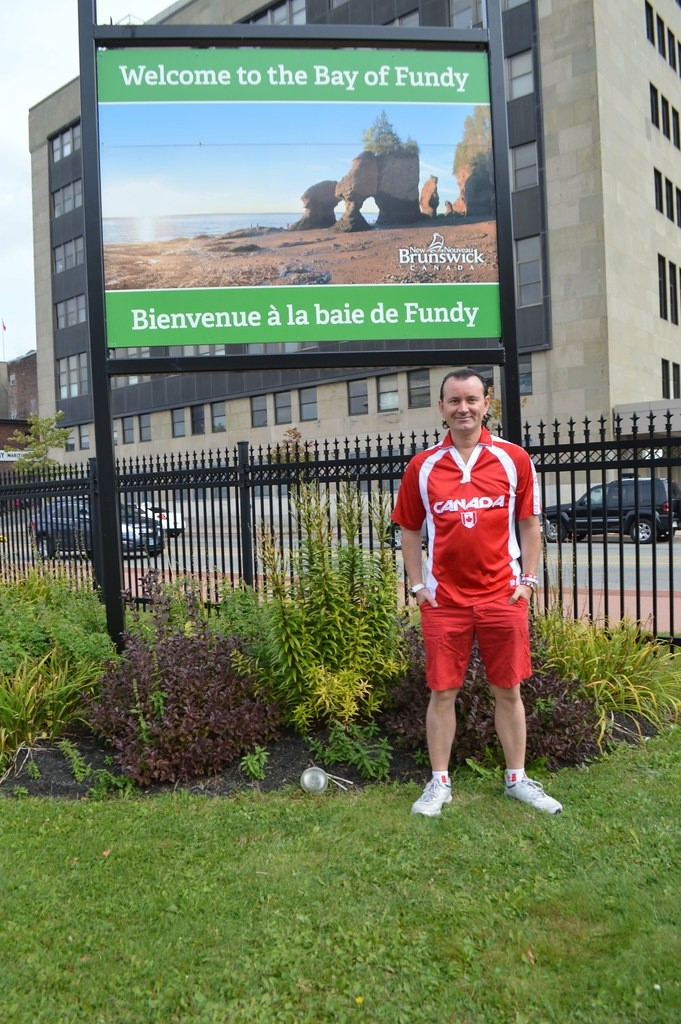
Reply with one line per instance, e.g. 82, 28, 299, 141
410, 778, 451, 816
505, 775, 562, 814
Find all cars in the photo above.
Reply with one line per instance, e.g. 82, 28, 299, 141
382, 514, 428, 551
28, 501, 164, 560
125, 502, 186, 538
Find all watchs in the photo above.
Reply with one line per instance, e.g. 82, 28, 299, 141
408, 583, 426, 597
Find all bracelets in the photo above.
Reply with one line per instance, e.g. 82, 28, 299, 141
520, 573, 539, 592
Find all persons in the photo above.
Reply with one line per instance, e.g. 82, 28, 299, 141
389, 369, 562, 820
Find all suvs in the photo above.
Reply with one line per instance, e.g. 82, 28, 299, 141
541, 478, 681, 543
